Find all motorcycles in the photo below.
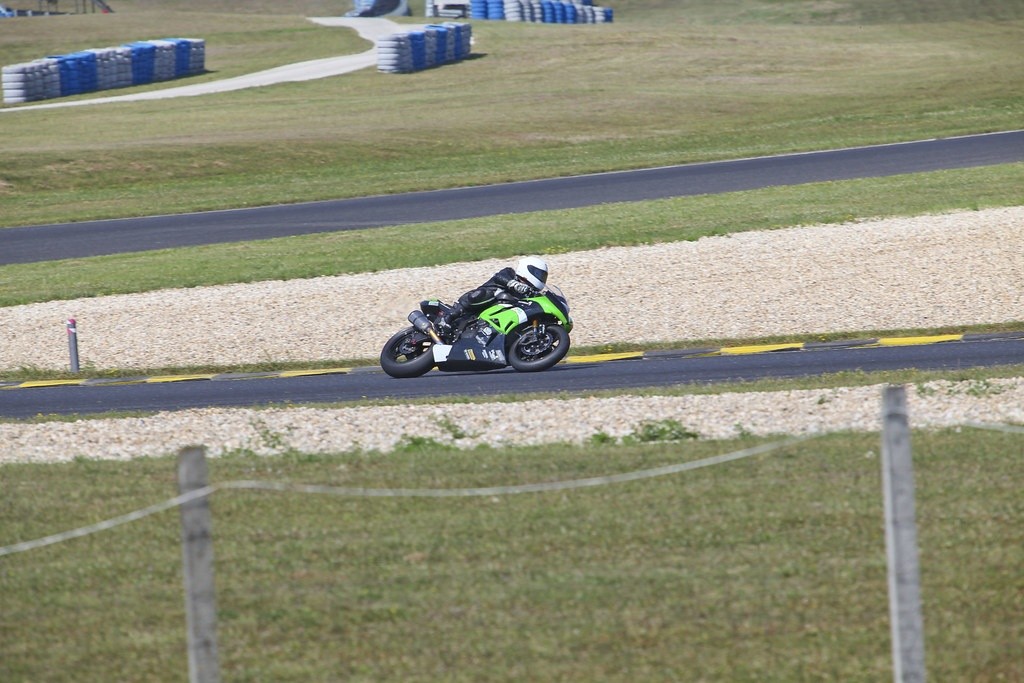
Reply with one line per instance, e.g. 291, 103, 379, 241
380, 285, 573, 378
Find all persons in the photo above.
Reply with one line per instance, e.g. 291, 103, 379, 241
434, 256, 548, 333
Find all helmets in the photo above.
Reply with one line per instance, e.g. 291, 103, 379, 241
516, 256, 548, 290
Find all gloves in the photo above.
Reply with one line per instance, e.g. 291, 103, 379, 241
506, 279, 529, 294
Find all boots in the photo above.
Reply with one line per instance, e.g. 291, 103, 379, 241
438, 301, 468, 336
